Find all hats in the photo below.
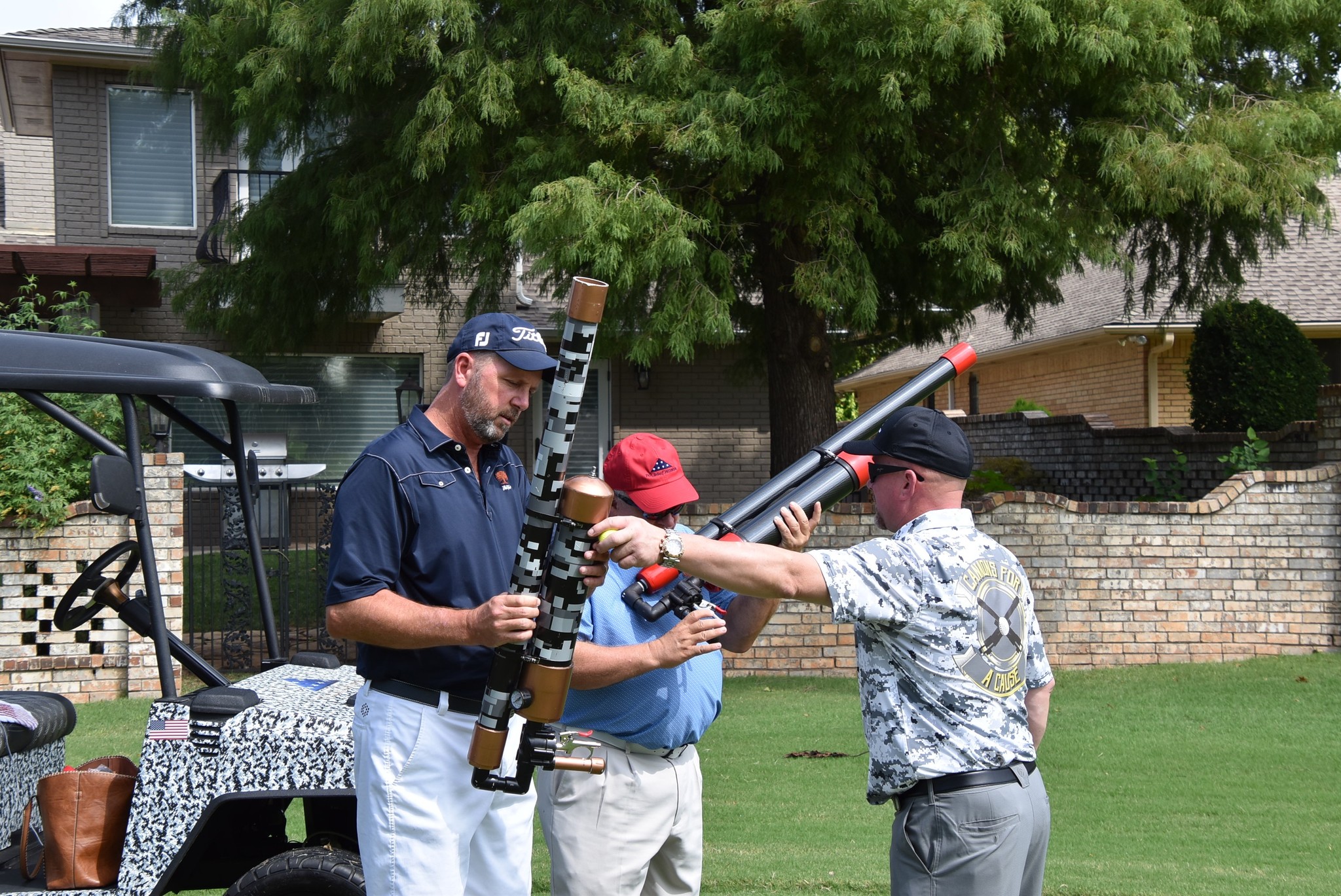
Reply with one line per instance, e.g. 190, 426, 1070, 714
447, 313, 559, 371
842, 406, 974, 478
602, 433, 699, 514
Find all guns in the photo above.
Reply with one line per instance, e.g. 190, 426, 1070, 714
468, 275, 618, 794
621, 339, 981, 644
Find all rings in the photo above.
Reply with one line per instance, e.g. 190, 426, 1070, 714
602, 563, 608, 576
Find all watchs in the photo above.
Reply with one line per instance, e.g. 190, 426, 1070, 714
659, 528, 685, 569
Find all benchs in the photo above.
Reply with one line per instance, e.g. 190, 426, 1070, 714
0, 690, 76, 850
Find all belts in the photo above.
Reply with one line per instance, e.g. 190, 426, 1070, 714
549, 722, 688, 759
365, 677, 515, 719
897, 760, 1036, 795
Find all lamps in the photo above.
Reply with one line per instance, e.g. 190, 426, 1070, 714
395, 372, 426, 426
635, 363, 651, 391
147, 394, 176, 452
1118, 336, 1147, 345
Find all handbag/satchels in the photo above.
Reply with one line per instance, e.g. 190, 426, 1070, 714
19, 756, 139, 889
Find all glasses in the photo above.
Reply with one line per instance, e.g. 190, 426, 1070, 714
868, 462, 925, 482
613, 491, 686, 521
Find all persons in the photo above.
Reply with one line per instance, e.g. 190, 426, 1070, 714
587, 404, 1055, 896
537, 433, 821, 896
324, 312, 610, 896
